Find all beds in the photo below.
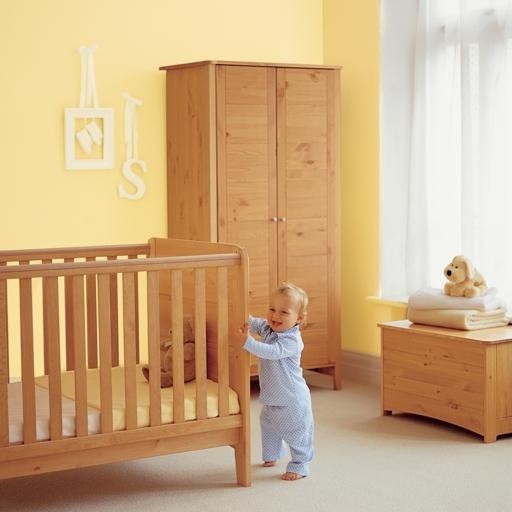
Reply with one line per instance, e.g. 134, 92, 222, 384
0, 237, 251, 487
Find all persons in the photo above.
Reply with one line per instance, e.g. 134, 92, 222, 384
239, 280, 315, 480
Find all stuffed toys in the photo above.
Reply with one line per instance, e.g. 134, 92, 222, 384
442, 253, 487, 298
139, 311, 196, 387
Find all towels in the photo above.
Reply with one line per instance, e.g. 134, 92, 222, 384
407, 310, 512, 330
408, 287, 504, 311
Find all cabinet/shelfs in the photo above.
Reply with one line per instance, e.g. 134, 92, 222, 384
160, 60, 344, 390
377, 320, 512, 443
